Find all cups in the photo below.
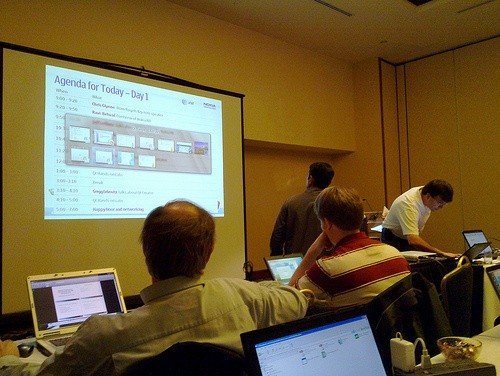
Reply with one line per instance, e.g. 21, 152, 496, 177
484, 250, 492, 265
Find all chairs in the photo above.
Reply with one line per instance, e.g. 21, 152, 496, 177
122, 342, 247, 376
364, 256, 474, 376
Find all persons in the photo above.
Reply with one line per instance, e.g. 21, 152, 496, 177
289, 186, 410, 310
381, 179, 462, 257
0, 200, 308, 376
270, 162, 335, 256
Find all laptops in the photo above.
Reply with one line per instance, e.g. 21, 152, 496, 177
26, 268, 127, 355
452, 243, 492, 262
238, 303, 394, 376
264, 253, 304, 286
462, 229, 500, 260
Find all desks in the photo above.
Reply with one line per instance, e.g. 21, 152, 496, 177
416, 323, 500, 376
15, 252, 463, 365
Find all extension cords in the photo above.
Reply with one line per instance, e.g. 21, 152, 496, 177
394, 363, 496, 376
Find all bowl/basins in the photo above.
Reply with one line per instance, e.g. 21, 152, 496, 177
437, 336, 482, 365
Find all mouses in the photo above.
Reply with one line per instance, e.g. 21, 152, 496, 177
17, 345, 34, 357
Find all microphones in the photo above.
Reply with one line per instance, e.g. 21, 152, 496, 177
362, 199, 375, 218
